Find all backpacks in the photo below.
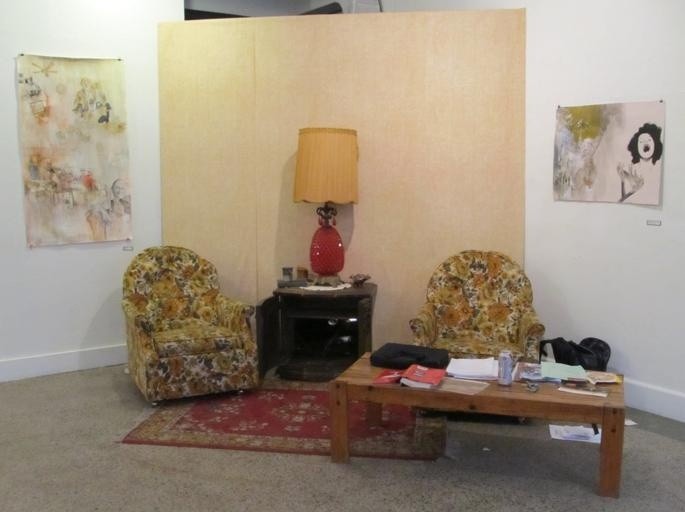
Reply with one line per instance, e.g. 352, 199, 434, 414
539, 337, 611, 372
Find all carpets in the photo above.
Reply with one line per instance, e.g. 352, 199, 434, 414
119, 386, 449, 463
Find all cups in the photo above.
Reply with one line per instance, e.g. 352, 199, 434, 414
523, 337, 540, 375
281, 265, 308, 282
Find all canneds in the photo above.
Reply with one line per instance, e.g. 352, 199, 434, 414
497, 350, 512, 385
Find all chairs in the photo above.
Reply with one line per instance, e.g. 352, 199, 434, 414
406, 245, 550, 427
120, 241, 263, 407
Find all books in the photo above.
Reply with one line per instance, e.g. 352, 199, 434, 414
371, 362, 408, 388
401, 365, 446, 390
445, 356, 623, 385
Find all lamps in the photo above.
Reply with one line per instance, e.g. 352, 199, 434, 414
290, 126, 363, 288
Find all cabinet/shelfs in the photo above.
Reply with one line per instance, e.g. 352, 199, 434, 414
266, 276, 378, 384
